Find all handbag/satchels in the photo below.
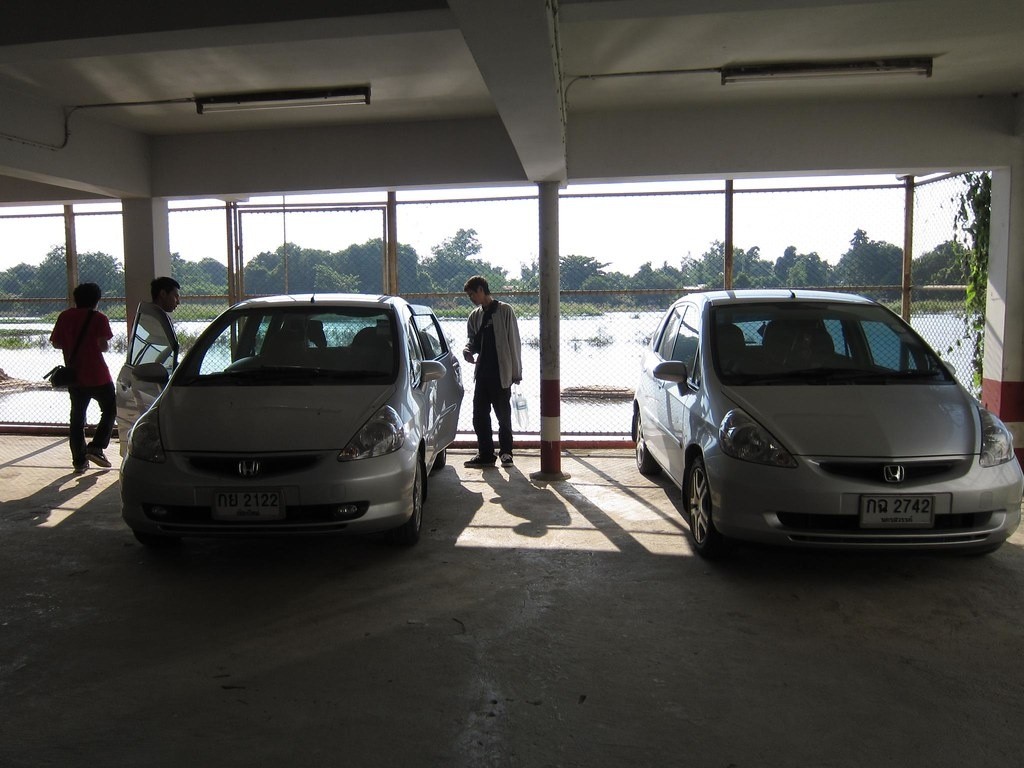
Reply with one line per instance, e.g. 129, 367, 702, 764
43, 365, 69, 389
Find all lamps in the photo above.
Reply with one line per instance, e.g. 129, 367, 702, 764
719, 57, 934, 87
197, 87, 370, 116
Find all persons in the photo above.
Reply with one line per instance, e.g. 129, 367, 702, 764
49, 283, 117, 472
150, 277, 181, 312
463, 276, 525, 467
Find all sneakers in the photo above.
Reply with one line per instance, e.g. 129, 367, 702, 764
501, 454, 514, 467
464, 456, 494, 468
75, 460, 89, 473
85, 443, 111, 467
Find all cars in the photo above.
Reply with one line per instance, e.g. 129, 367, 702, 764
632, 288, 1022, 558
115, 293, 465, 547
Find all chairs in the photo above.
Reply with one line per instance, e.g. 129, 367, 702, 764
714, 326, 758, 376
267, 329, 306, 364
351, 328, 390, 361
792, 330, 836, 369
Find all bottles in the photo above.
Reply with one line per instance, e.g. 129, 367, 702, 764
516, 393, 529, 428
512, 391, 518, 420
